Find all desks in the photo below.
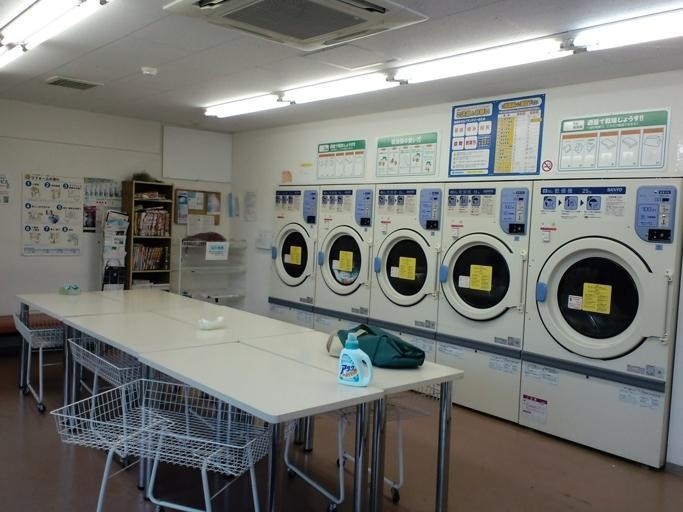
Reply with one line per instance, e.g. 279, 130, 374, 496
239, 331, 464, 512
138, 342, 385, 512
96, 289, 222, 312
62, 312, 240, 491
16, 292, 152, 424
151, 306, 316, 453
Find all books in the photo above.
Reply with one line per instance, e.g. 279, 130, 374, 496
134, 244, 170, 272
103, 210, 129, 289
135, 188, 172, 200
133, 206, 172, 237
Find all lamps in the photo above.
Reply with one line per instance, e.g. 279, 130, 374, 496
0, 0, 110, 52
0, 44, 28, 68
282, 72, 400, 104
573, 8, 683, 52
204, 94, 291, 118
394, 38, 576, 85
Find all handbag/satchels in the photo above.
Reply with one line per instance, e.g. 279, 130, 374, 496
326, 322, 425, 369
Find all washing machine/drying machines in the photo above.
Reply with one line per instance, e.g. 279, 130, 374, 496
368, 185, 448, 401
267, 185, 318, 330
518, 180, 683, 470
313, 185, 374, 338
434, 180, 534, 427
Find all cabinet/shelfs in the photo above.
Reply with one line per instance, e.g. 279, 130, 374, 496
121, 181, 175, 293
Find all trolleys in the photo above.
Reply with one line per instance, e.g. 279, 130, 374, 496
12, 309, 65, 414
65, 336, 189, 469
284, 382, 442, 511
49, 336, 272, 512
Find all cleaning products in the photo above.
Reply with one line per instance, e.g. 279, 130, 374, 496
338, 333, 373, 388
